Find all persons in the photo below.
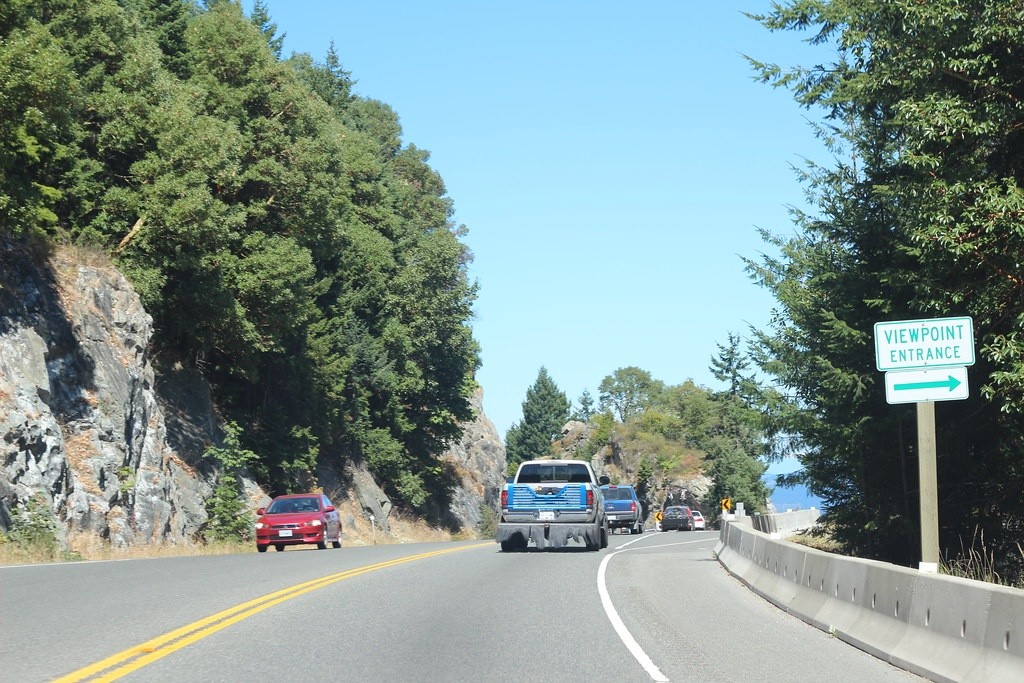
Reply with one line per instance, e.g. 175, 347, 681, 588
311, 499, 318, 508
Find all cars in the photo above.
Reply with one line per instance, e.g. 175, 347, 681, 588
255, 494, 342, 552
661, 506, 706, 532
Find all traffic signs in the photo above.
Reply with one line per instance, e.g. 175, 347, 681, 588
873, 317, 975, 405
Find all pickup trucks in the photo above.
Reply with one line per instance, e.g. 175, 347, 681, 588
496, 460, 610, 552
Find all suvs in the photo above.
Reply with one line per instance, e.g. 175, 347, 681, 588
600, 483, 646, 534
